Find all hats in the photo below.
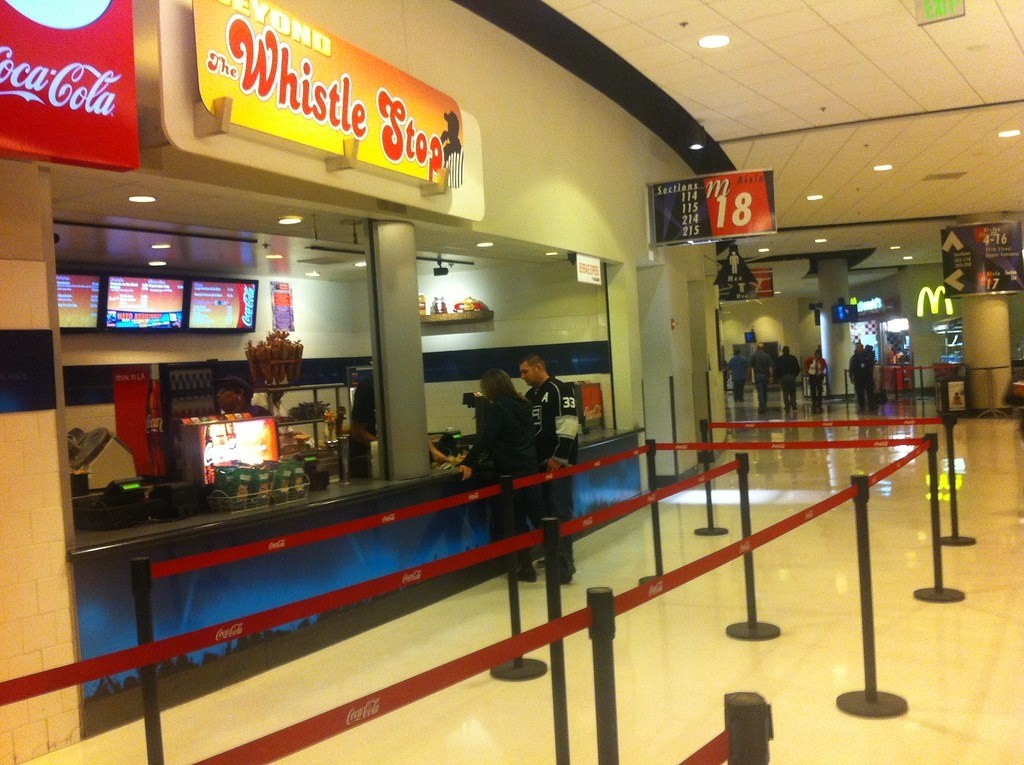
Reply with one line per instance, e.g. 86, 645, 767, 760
216, 376, 254, 397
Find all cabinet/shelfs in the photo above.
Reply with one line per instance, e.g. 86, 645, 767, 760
254, 383, 348, 483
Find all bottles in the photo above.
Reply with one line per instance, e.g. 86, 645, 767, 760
192, 374, 200, 400
177, 375, 185, 400
206, 374, 212, 398
184, 374, 193, 401
419, 294, 426, 316
199, 373, 206, 399
146, 364, 167, 478
171, 375, 177, 401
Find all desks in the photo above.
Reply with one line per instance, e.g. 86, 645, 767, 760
971, 366, 1010, 419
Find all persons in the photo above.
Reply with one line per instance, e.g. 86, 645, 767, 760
520, 353, 582, 585
892, 343, 904, 358
749, 343, 775, 412
864, 345, 875, 372
772, 346, 800, 411
458, 368, 546, 583
848, 343, 880, 413
350, 375, 448, 479
728, 349, 748, 402
804, 349, 827, 406
723, 360, 730, 389
213, 377, 280, 457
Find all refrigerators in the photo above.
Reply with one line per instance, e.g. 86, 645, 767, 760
111, 360, 225, 488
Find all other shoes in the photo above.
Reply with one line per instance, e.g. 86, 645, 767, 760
535, 557, 543, 568
518, 567, 537, 583
561, 558, 576, 585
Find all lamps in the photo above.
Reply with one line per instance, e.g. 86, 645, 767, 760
433, 260, 448, 276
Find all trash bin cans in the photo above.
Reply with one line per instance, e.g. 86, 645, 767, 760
935, 378, 969, 413
